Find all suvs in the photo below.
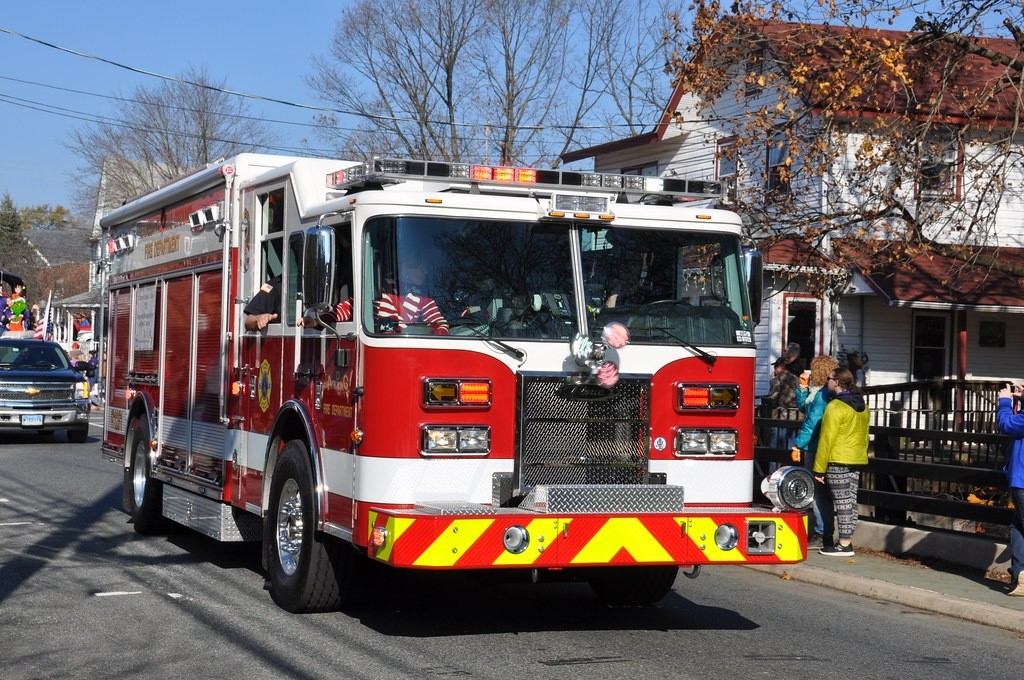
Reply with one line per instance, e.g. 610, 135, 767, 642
0, 337, 90, 442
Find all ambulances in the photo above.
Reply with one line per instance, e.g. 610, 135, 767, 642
92, 150, 816, 613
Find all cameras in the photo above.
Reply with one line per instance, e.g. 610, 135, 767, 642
1009, 385, 1016, 392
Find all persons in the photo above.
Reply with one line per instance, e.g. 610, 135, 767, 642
66, 342, 100, 408
14, 348, 51, 367
297, 244, 451, 337
812, 368, 870, 556
782, 342, 805, 377
763, 357, 800, 473
101, 352, 107, 406
242, 270, 283, 330
792, 355, 841, 550
0, 284, 22, 331
851, 351, 869, 388
604, 244, 670, 311
997, 383, 1024, 597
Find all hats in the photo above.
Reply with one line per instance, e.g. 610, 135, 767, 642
770, 356, 788, 366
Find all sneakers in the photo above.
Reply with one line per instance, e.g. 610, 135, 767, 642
818, 541, 855, 559
1003, 569, 1023, 598
804, 536, 834, 550
